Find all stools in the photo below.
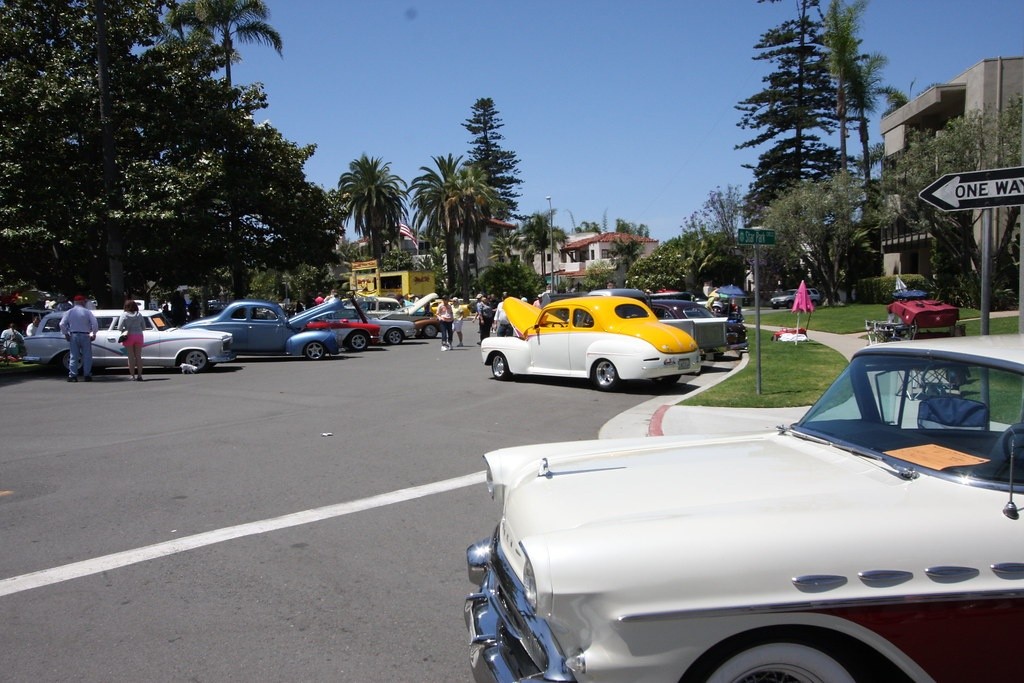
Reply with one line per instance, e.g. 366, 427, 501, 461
864, 319, 916, 343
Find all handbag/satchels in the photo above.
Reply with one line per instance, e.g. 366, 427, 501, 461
118, 329, 128, 343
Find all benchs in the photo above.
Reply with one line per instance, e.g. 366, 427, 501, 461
0, 337, 10, 366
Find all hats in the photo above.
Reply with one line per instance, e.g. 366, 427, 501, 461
453, 297, 458, 302
476, 294, 484, 299
73, 295, 87, 301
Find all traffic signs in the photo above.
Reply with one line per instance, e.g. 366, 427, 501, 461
919, 167, 1024, 211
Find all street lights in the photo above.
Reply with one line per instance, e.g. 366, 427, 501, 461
546, 195, 555, 296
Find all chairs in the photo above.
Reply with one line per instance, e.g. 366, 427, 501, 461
915, 396, 989, 431
986, 422, 1024, 486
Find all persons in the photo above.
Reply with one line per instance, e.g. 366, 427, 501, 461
163, 291, 201, 327
117, 300, 146, 381
296, 289, 347, 319
436, 295, 464, 351
395, 294, 431, 316
59, 296, 98, 382
473, 284, 556, 345
607, 280, 616, 289
0, 316, 41, 362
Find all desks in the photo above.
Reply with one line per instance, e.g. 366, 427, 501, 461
891, 288, 928, 302
887, 300, 960, 338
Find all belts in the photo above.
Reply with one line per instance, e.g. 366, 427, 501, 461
70, 331, 89, 334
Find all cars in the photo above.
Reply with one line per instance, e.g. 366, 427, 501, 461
21, 309, 237, 376
478, 296, 703, 393
354, 291, 443, 339
771, 289, 820, 309
539, 289, 749, 358
461, 332, 1023, 683
302, 292, 381, 353
318, 305, 418, 346
420, 299, 470, 319
179, 299, 345, 361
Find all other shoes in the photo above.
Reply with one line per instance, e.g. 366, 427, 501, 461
85, 376, 92, 381
137, 376, 143, 381
456, 342, 463, 347
127, 376, 135, 381
67, 377, 78, 382
441, 344, 453, 351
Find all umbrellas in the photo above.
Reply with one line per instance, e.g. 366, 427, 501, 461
791, 279, 813, 346
0, 287, 38, 305
895, 275, 909, 293
